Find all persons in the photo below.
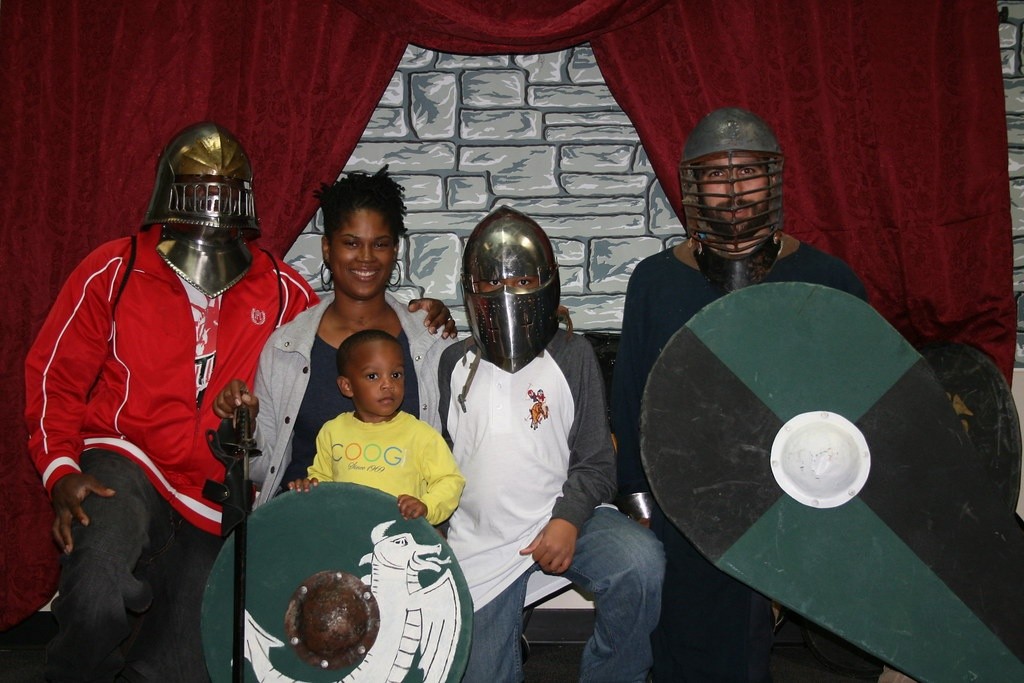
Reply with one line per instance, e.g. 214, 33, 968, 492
212, 163, 454, 511
613, 108, 867, 683
438, 206, 666, 683
26, 121, 457, 682
289, 329, 466, 524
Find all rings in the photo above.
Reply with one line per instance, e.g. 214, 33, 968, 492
448, 317, 455, 326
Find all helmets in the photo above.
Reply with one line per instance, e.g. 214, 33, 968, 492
461, 203, 560, 374
140, 121, 261, 298
678, 106, 783, 257
